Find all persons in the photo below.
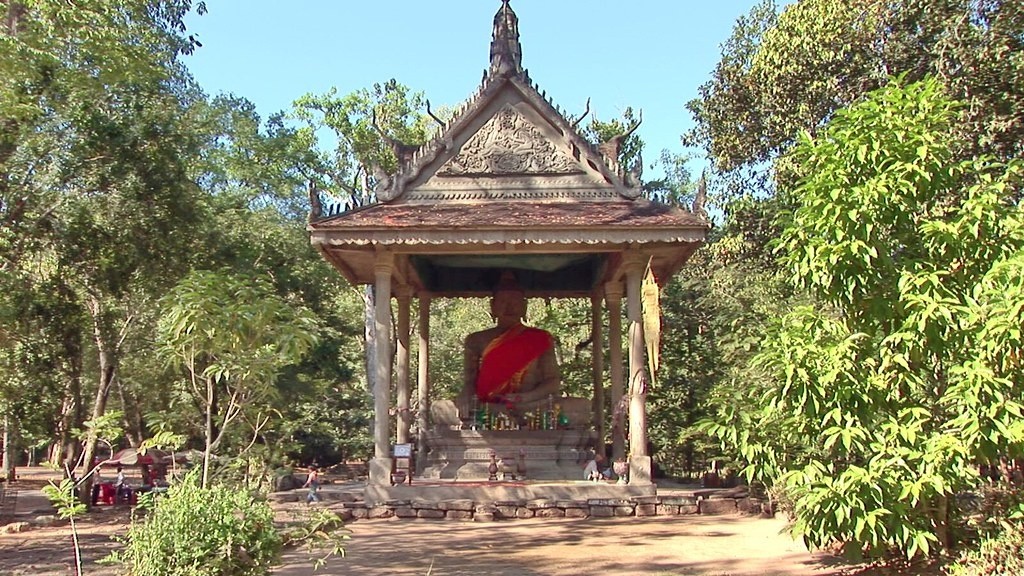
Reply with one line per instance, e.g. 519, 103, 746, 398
302, 465, 323, 503
430, 270, 592, 430
112, 467, 125, 505
92, 467, 104, 506
583, 453, 613, 480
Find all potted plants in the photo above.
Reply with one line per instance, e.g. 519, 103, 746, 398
391, 471, 406, 487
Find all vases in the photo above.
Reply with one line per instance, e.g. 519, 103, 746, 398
613, 462, 628, 484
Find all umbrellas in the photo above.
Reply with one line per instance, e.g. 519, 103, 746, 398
162, 447, 218, 461
106, 447, 173, 483
